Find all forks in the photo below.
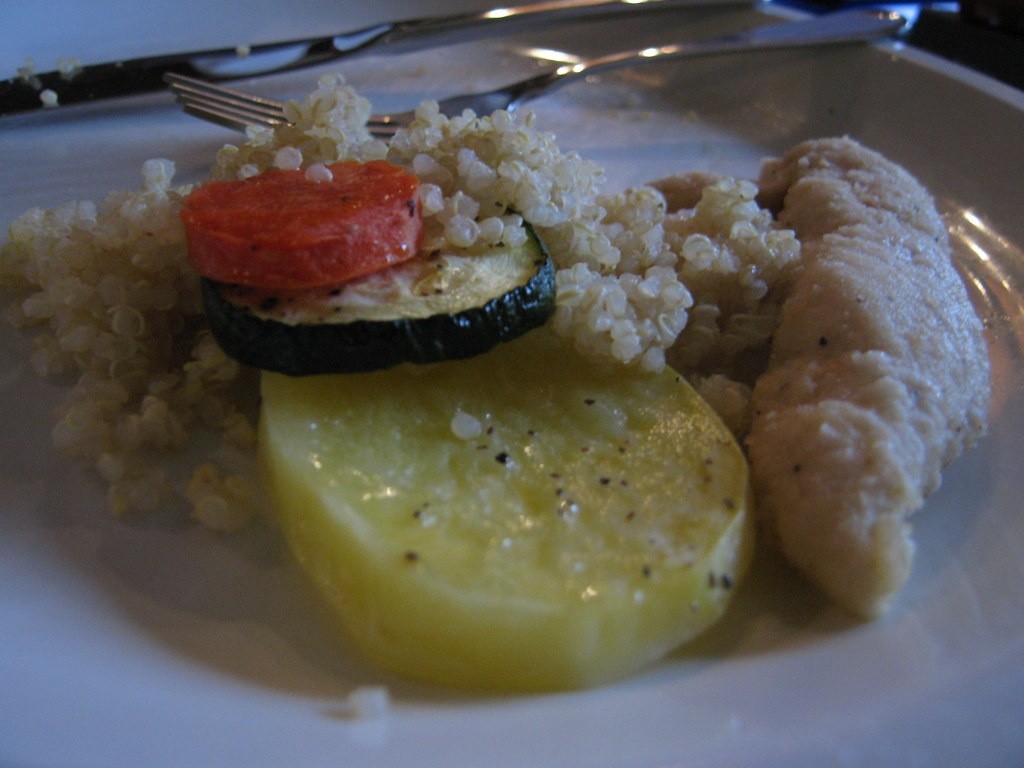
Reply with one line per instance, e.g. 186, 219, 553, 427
162, 5, 915, 147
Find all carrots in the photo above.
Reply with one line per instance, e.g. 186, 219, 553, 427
180, 160, 425, 289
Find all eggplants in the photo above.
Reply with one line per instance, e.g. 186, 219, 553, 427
200, 205, 556, 376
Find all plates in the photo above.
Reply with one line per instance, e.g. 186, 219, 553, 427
0, 0, 1022, 766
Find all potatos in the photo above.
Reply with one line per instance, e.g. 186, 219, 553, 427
253, 330, 756, 687
